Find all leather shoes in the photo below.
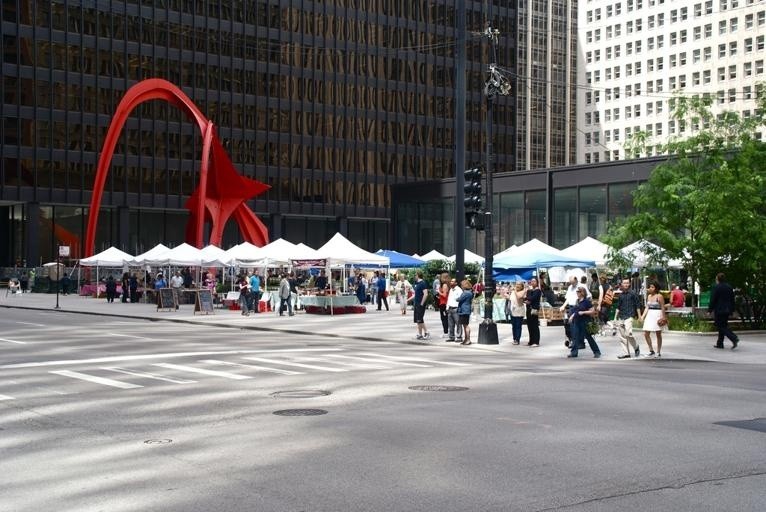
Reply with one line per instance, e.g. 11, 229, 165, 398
732, 337, 740, 350
712, 345, 724, 349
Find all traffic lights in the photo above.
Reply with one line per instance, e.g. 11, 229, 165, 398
466, 212, 479, 230
474, 169, 483, 210
463, 166, 473, 210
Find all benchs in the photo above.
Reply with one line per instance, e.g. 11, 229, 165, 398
696, 307, 741, 321
664, 306, 692, 322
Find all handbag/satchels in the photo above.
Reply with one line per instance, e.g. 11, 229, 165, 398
530, 309, 538, 315
587, 321, 600, 334
658, 318, 668, 325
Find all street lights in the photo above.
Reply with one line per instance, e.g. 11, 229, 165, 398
478, 65, 512, 346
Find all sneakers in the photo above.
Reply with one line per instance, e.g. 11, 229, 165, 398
416, 333, 662, 358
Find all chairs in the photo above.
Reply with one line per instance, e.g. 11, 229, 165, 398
4, 277, 21, 299
223, 290, 300, 312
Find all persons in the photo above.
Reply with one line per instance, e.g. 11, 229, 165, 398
706, 272, 741, 351
143, 270, 235, 310
348, 269, 389, 311
28, 268, 37, 291
62, 272, 69, 295
101, 271, 138, 303
394, 271, 554, 348
237, 270, 327, 317
560, 270, 685, 358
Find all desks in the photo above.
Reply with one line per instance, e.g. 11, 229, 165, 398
479, 298, 528, 323
300, 295, 361, 315
79, 284, 196, 302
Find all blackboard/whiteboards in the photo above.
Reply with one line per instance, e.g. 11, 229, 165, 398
159, 288, 175, 309
196, 289, 214, 312
